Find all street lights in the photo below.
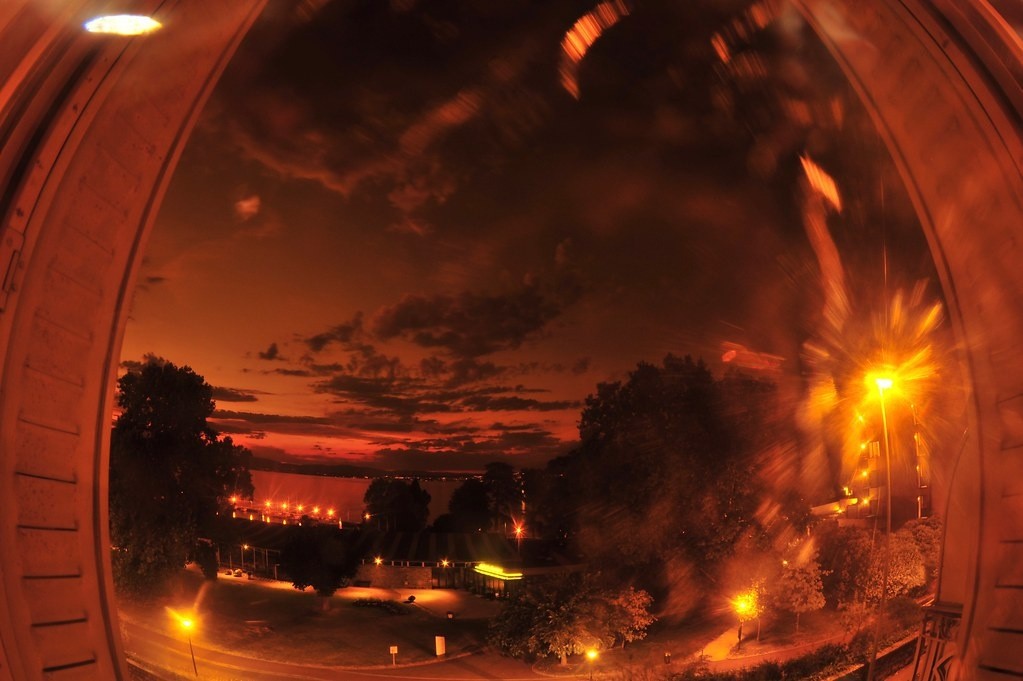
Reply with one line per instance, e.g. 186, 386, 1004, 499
861, 374, 899, 681
